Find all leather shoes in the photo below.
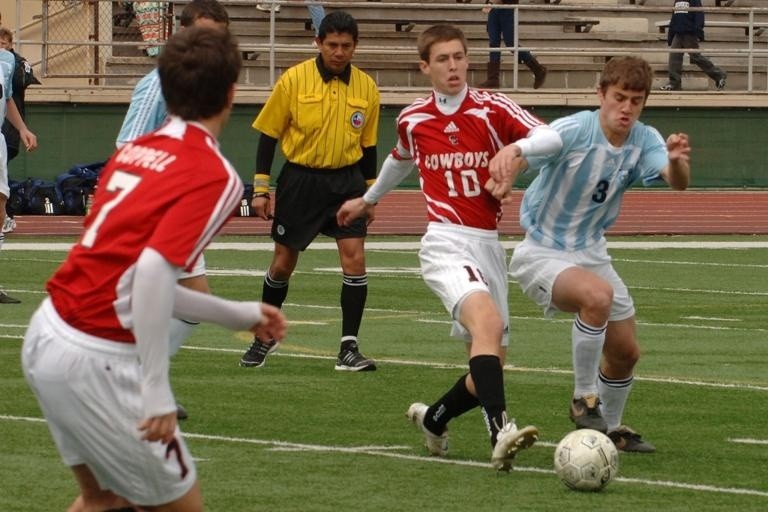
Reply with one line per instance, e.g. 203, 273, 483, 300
1, 291, 20, 303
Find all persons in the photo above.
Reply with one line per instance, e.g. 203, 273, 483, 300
475, 0, 547, 89
0, 29, 42, 233
307, 1, 326, 49
0, 9, 38, 304
20, 25, 287, 511
658, 0, 728, 91
336, 25, 564, 470
484, 55, 691, 453
239, 13, 381, 373
114, 1, 231, 416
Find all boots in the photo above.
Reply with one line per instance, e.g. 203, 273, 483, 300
477, 61, 500, 88
526, 56, 547, 89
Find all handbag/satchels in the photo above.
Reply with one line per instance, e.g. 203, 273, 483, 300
5, 161, 107, 220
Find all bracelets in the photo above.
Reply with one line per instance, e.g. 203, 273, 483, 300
253, 173, 271, 193
366, 178, 376, 191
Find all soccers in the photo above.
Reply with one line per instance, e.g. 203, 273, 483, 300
555, 429, 618, 492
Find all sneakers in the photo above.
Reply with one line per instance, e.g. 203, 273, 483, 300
2, 218, 14, 233
659, 84, 682, 90
490, 411, 539, 473
405, 402, 449, 457
607, 424, 656, 453
238, 334, 279, 368
715, 73, 727, 91
334, 342, 377, 371
568, 393, 608, 434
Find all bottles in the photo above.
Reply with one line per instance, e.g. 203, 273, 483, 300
85, 194, 95, 215
240, 199, 250, 218
44, 196, 54, 218
